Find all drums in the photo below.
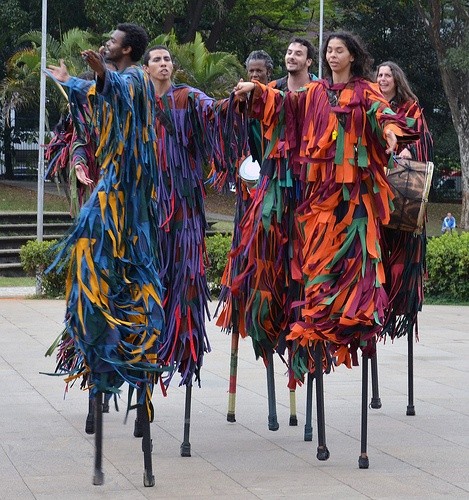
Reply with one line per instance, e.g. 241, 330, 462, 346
239, 155, 260, 195
380, 158, 434, 235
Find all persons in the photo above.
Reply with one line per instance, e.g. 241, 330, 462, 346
40, 39, 433, 407
442, 213, 455, 234
235, 34, 421, 388
45, 23, 174, 406
